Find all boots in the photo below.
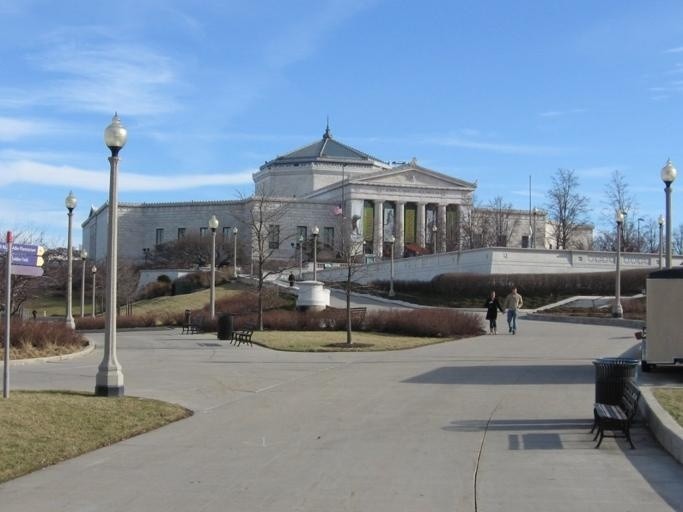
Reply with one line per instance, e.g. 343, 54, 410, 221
488, 327, 496, 335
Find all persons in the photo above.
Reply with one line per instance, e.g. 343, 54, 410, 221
287, 271, 295, 286
483, 289, 504, 335
501, 285, 523, 335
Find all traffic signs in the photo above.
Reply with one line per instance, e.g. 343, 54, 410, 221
10, 252, 44, 266
10, 243, 46, 257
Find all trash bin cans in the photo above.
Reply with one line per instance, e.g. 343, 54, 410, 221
215, 312, 238, 340
595, 357, 641, 361
591, 360, 642, 405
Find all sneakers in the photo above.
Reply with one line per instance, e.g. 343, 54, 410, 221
509, 327, 515, 335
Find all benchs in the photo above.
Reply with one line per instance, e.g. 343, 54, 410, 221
229, 320, 256, 347
589, 381, 642, 451
180, 315, 204, 335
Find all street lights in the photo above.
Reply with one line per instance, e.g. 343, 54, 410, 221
386, 232, 398, 299
86, 262, 97, 319
530, 206, 537, 249
294, 232, 304, 279
205, 214, 222, 324
430, 224, 437, 253
61, 189, 80, 331
76, 245, 88, 320
609, 153, 675, 325
308, 221, 320, 282
228, 224, 241, 278
89, 110, 129, 397
